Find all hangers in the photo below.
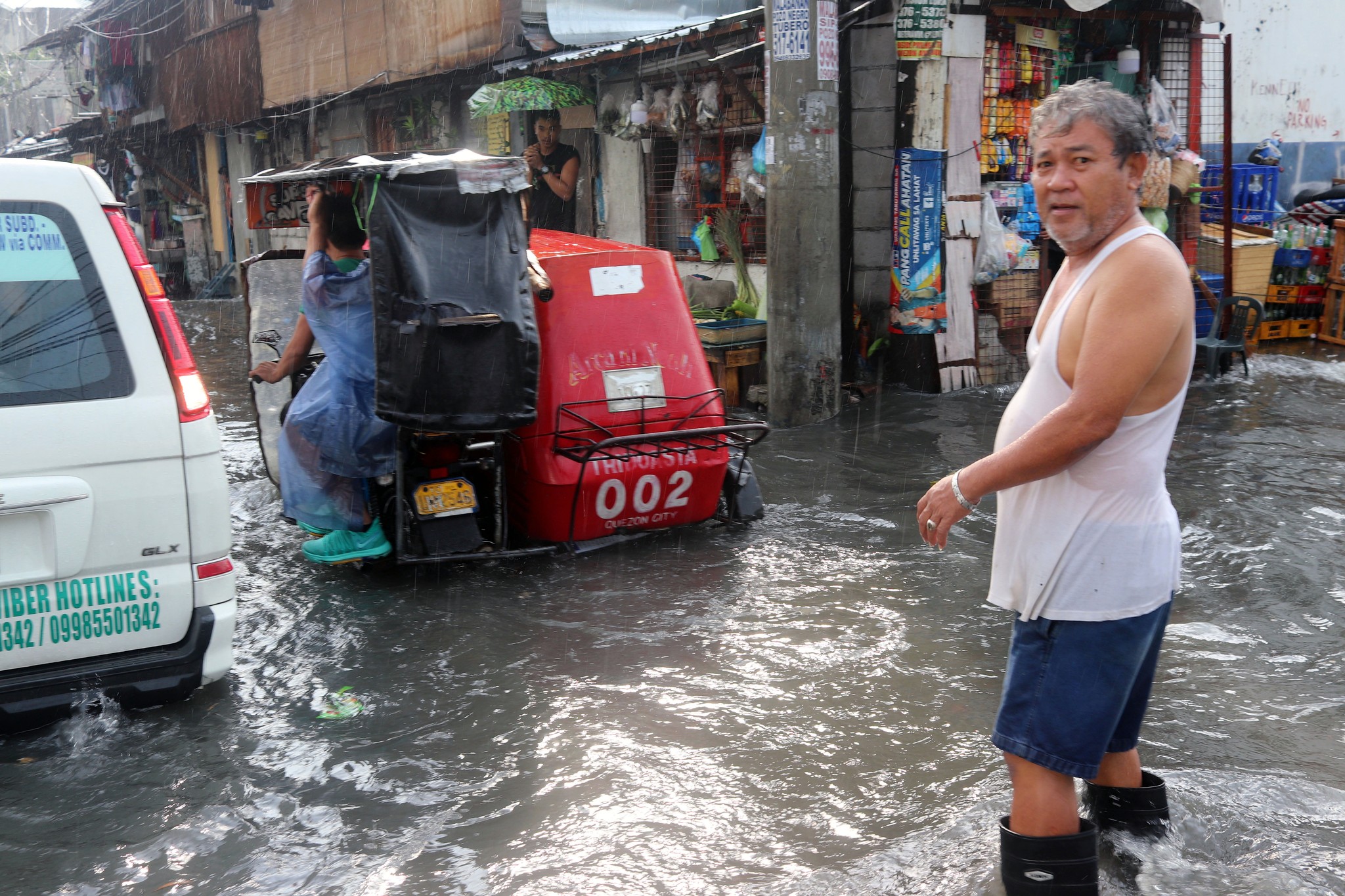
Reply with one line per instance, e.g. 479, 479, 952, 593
64, 51, 84, 70
73, 82, 89, 93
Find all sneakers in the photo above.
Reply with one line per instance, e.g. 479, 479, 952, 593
302, 516, 392, 565
296, 504, 350, 536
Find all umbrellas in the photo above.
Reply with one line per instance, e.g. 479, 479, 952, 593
466, 75, 598, 150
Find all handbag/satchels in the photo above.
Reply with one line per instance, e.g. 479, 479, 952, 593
752, 124, 767, 176
970, 192, 1034, 288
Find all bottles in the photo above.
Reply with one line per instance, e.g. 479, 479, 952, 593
1218, 174, 1335, 330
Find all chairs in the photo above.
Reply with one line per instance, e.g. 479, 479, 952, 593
1196, 296, 1263, 381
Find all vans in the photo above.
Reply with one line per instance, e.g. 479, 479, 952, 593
0, 150, 238, 747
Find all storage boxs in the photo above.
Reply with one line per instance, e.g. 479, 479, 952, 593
694, 318, 767, 345
983, 251, 1040, 349
1260, 245, 1333, 340
1192, 224, 1280, 337
1200, 163, 1280, 223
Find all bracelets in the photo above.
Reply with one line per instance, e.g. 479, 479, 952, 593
952, 469, 981, 509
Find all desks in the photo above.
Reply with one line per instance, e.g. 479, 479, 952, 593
148, 249, 184, 262
701, 340, 766, 408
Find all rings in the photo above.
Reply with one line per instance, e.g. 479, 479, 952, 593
926, 519, 938, 531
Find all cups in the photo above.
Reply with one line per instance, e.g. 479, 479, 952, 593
641, 138, 652, 153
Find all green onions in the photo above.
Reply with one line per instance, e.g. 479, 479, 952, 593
687, 292, 728, 320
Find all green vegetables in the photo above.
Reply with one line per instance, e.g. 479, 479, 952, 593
712, 208, 760, 307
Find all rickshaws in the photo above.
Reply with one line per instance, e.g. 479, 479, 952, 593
235, 146, 771, 571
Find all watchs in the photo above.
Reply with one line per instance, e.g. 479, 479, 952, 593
538, 165, 550, 175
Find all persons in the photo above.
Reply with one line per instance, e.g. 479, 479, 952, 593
248, 186, 396, 564
917, 77, 1197, 896
219, 166, 237, 262
521, 109, 582, 234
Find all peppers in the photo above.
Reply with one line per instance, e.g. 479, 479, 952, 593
723, 300, 757, 319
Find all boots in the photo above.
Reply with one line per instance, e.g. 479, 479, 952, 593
1083, 768, 1173, 867
998, 814, 1099, 896
